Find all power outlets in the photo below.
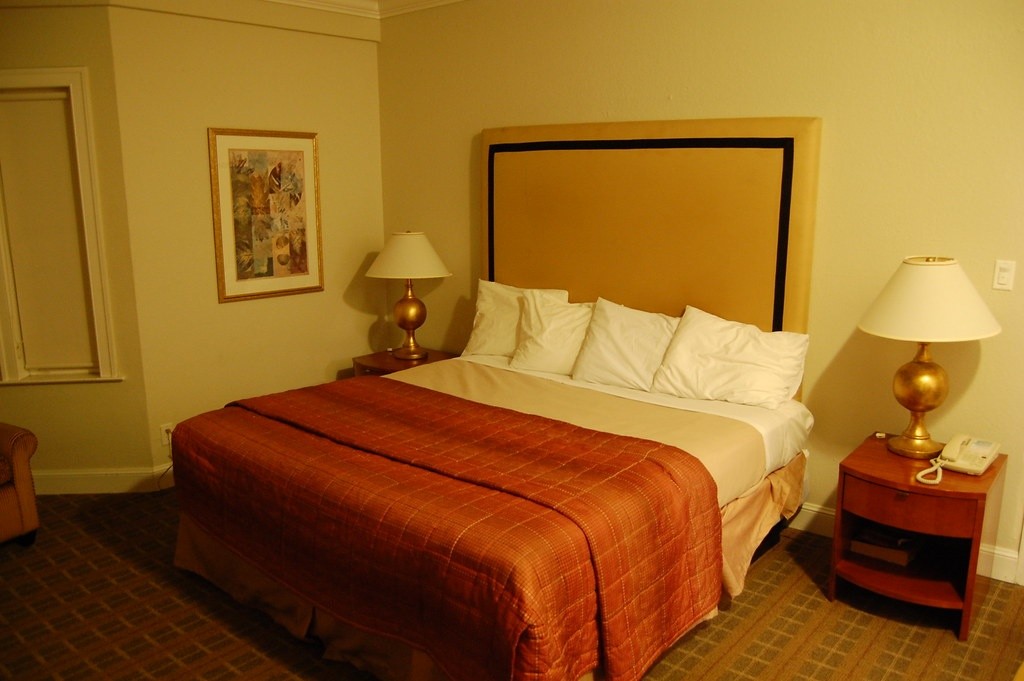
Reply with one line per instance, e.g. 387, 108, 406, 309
160, 422, 173, 446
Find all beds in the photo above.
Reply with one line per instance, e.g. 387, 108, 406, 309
169, 118, 818, 681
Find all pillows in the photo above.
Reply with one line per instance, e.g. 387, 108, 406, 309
510, 290, 597, 376
571, 297, 680, 392
652, 305, 811, 414
460, 279, 568, 358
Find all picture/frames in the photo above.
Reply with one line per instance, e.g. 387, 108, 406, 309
206, 127, 325, 305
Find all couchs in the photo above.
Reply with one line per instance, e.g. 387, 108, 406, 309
0, 423, 40, 547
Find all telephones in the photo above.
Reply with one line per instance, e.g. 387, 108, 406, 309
935, 434, 1001, 477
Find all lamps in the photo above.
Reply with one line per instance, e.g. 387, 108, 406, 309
363, 230, 452, 360
857, 257, 1003, 460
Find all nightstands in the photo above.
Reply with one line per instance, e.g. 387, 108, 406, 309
828, 430, 1008, 642
353, 347, 454, 378
